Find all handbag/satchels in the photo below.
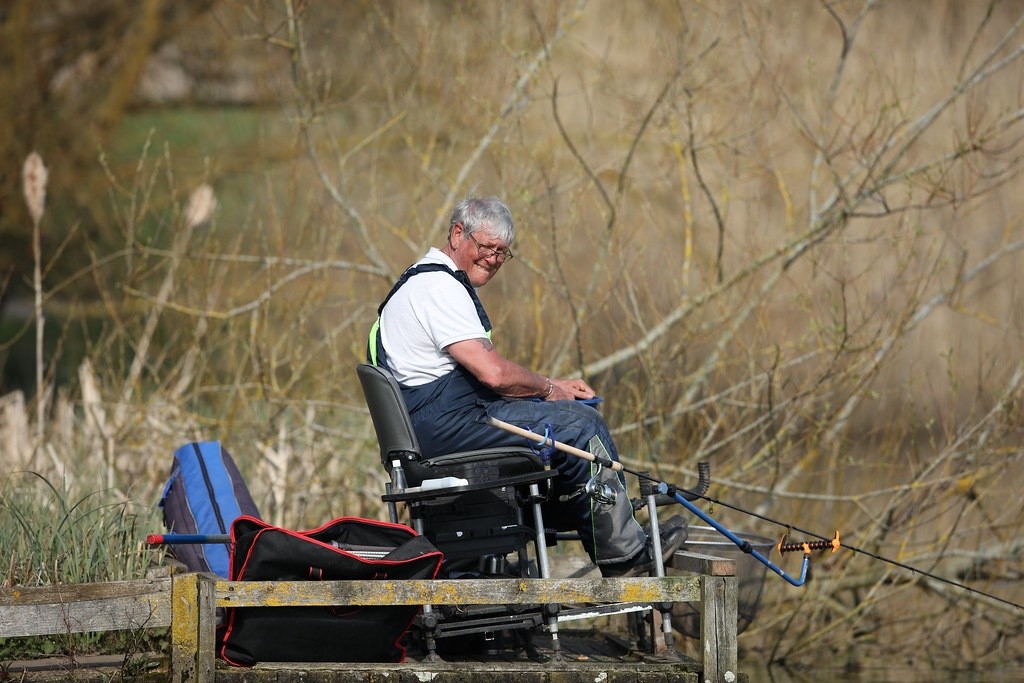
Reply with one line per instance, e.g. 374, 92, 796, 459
216, 515, 444, 667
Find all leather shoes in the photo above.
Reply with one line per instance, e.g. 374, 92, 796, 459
590, 515, 687, 566
598, 527, 689, 578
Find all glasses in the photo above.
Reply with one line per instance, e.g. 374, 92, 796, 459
465, 229, 513, 263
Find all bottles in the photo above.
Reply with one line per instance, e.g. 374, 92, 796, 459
390, 459, 408, 496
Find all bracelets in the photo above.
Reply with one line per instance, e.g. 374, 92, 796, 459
541, 378, 553, 397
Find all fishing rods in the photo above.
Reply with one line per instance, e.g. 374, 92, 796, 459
475, 415, 1024, 614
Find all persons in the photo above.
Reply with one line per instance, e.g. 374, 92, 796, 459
374, 196, 689, 578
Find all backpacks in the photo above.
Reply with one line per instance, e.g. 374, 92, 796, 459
158, 441, 263, 616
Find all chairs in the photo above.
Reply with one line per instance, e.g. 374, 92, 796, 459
353, 363, 566, 670
